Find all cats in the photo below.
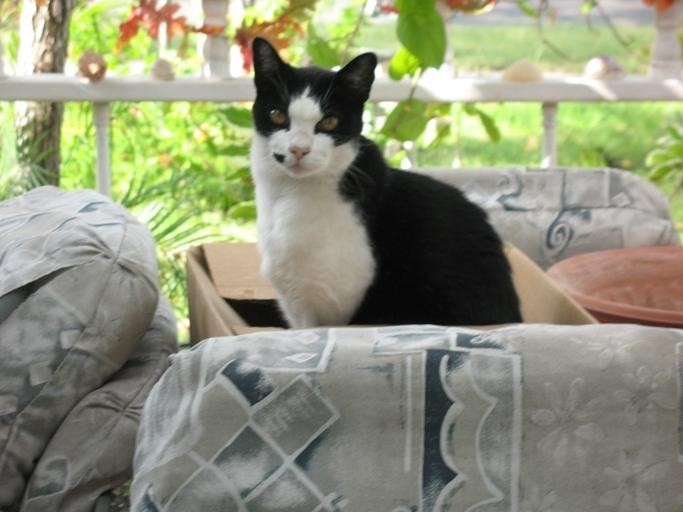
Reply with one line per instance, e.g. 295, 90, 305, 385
245, 35, 524, 329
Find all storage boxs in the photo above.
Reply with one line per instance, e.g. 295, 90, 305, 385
183, 236, 603, 347
